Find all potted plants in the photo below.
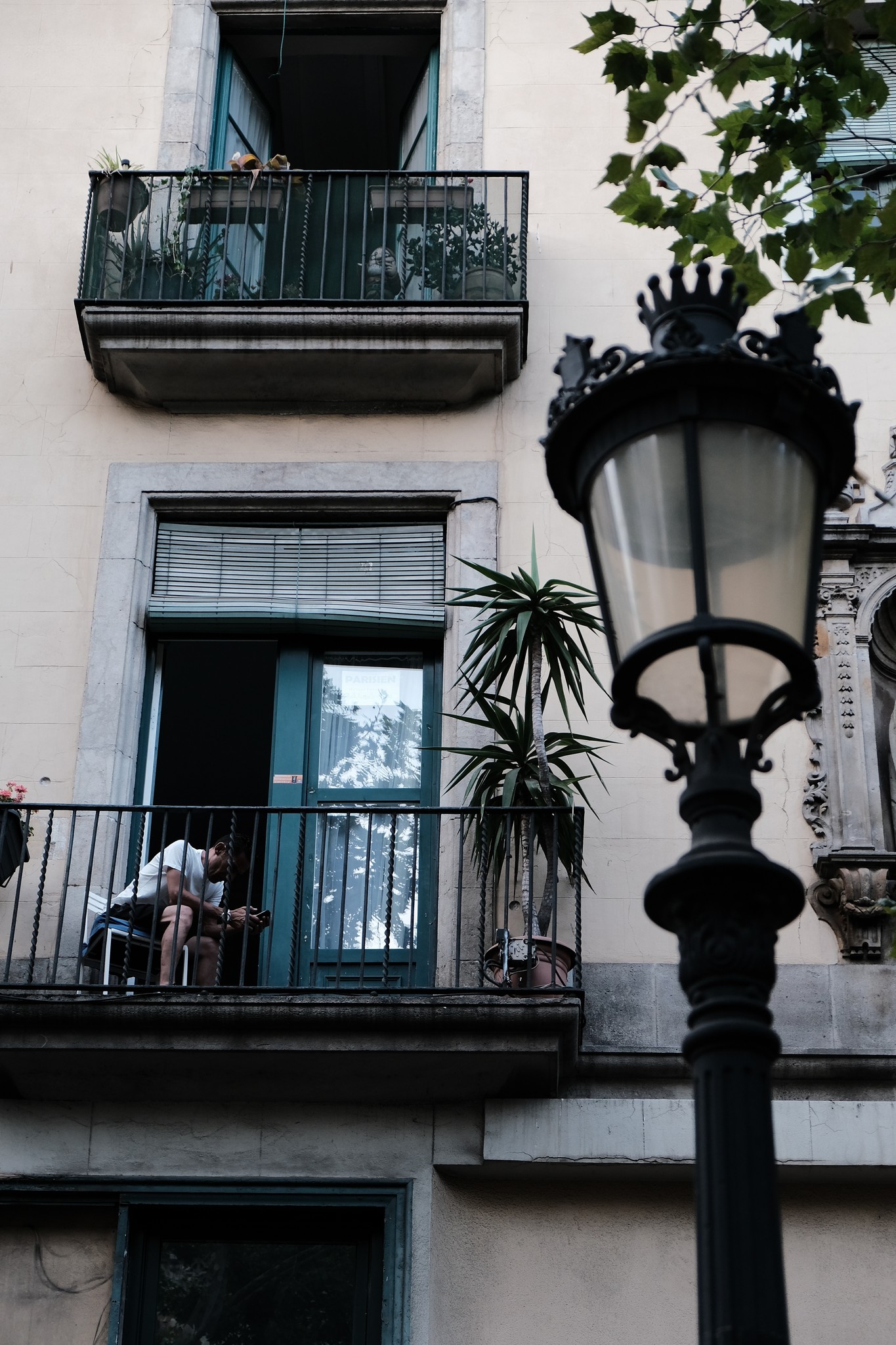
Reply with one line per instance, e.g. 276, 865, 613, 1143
400, 202, 522, 300
421, 523, 625, 998
96, 212, 228, 299
167, 155, 306, 269
84, 143, 152, 235
366, 174, 476, 226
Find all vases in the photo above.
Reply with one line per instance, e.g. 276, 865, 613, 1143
0, 809, 30, 889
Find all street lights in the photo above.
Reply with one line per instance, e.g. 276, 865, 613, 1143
538, 260, 859, 1345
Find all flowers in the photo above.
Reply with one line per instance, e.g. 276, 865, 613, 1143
0, 780, 50, 842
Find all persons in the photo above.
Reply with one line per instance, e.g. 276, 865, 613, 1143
88, 834, 271, 992
357, 247, 400, 299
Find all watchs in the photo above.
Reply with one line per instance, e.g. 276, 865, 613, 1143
220, 909, 231, 924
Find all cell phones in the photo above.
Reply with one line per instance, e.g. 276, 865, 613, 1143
254, 910, 271, 921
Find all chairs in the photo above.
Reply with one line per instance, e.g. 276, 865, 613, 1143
76, 884, 190, 995
